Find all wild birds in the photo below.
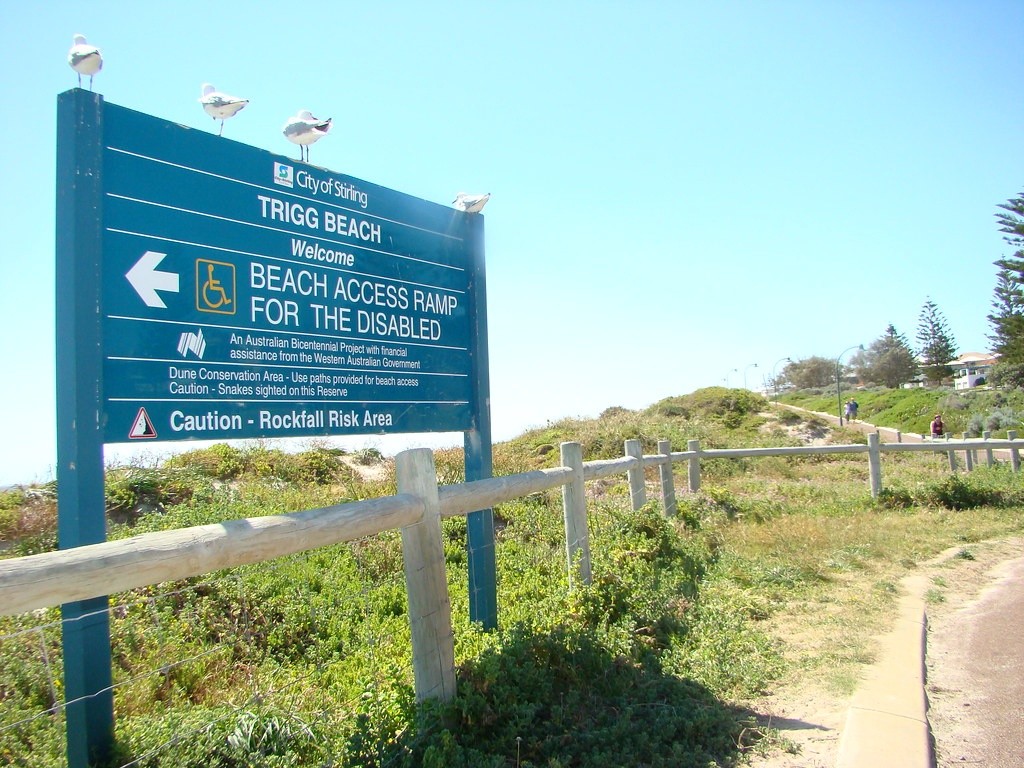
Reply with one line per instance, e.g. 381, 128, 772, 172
69, 34, 103, 92
451, 192, 491, 213
282, 109, 332, 163
199, 83, 250, 137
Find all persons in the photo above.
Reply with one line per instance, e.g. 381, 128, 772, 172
930, 415, 947, 437
844, 398, 858, 424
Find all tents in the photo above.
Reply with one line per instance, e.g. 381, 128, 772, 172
943, 351, 1002, 365
